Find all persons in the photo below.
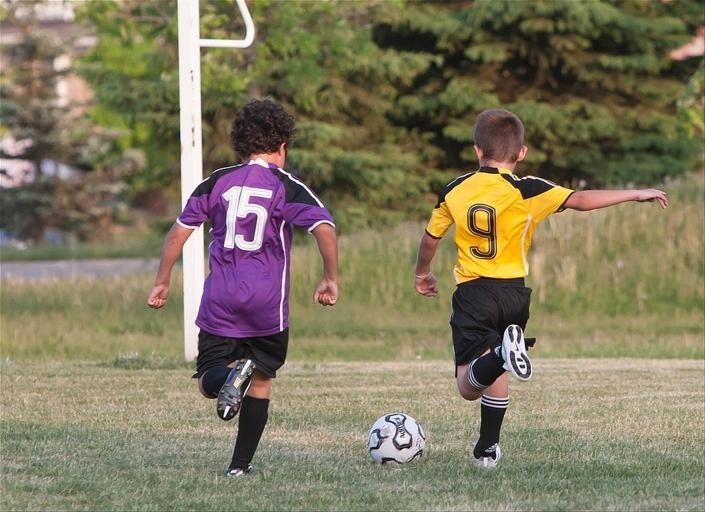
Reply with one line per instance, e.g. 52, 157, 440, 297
142, 94, 341, 483
410, 105, 674, 476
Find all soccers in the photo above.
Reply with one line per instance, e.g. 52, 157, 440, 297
368, 413, 426, 466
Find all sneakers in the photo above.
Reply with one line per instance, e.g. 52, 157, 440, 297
501, 324, 533, 381
474, 443, 500, 469
216, 359, 256, 422
227, 461, 252, 478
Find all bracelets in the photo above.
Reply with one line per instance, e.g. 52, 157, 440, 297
414, 271, 433, 281
